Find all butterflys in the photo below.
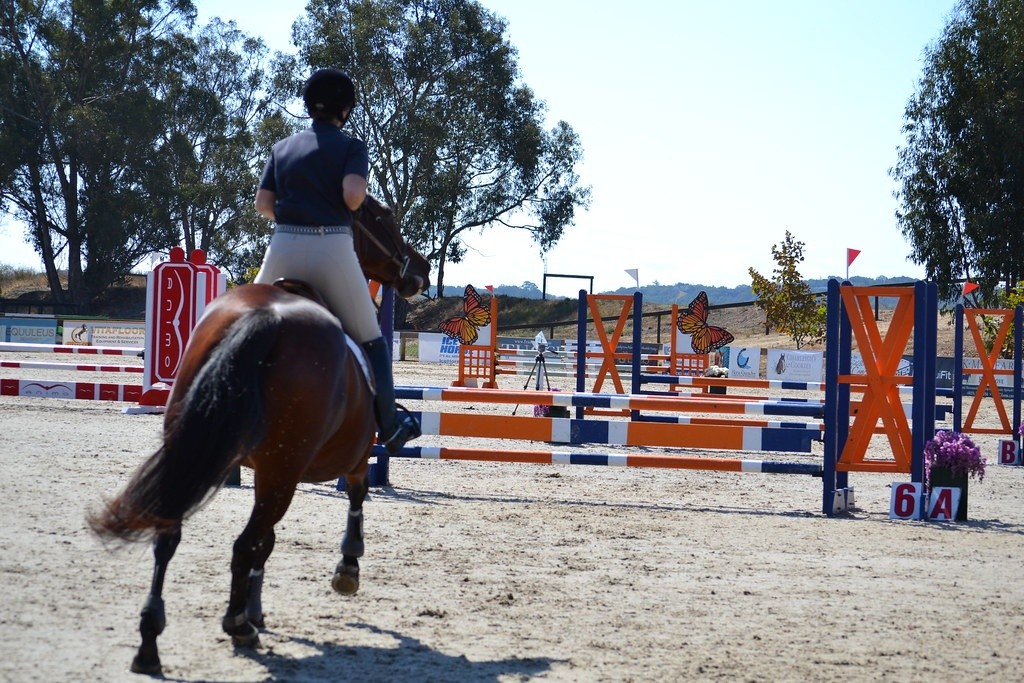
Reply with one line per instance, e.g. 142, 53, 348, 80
677, 291, 734, 354
438, 284, 491, 346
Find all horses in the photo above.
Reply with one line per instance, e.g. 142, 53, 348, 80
85, 194, 431, 674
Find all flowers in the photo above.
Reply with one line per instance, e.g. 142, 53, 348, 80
1018, 422, 1024, 436
923, 431, 988, 485
704, 365, 729, 378
534, 387, 561, 416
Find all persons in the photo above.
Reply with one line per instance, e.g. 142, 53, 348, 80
252, 67, 422, 454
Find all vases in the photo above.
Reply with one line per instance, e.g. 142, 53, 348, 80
549, 406, 565, 418
929, 469, 968, 521
710, 373, 726, 395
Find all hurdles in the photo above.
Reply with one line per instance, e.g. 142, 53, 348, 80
0, 249, 1022, 525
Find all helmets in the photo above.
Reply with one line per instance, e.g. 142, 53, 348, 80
303, 69, 356, 111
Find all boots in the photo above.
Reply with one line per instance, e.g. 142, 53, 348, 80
359, 337, 419, 452
